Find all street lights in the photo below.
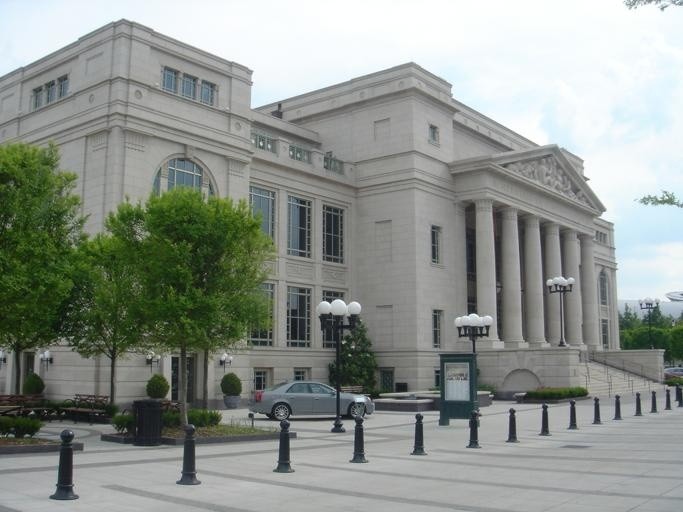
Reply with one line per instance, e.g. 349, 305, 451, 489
316, 295, 363, 434
638, 297, 662, 350
453, 311, 494, 431
544, 275, 575, 349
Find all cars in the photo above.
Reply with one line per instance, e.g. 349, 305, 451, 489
249, 379, 376, 425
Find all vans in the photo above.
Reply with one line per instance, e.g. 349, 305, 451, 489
663, 367, 683, 381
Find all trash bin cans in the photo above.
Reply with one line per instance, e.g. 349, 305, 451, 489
131, 399, 163, 446
